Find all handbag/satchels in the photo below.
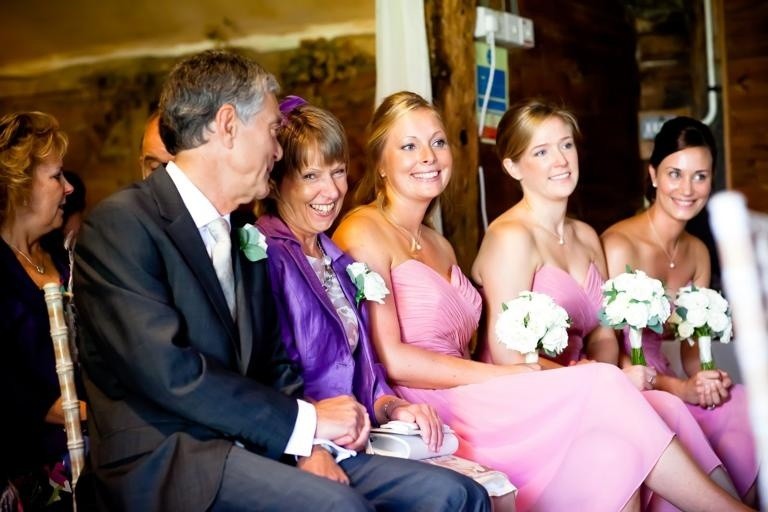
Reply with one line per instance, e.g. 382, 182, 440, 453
366, 420, 459, 460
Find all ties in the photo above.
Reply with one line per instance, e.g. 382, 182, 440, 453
209, 219, 237, 323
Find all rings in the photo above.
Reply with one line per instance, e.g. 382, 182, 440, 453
707, 404, 715, 411
648, 377, 653, 383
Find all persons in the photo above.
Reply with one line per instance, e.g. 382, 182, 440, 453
0, 110, 90, 512
253, 96, 519, 512
330, 91, 757, 512
600, 116, 759, 512
139, 108, 175, 181
471, 98, 743, 512
42, 171, 86, 246
70, 50, 492, 512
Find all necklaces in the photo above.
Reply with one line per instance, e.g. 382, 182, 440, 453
647, 208, 679, 269
380, 206, 422, 249
16, 246, 45, 274
523, 200, 564, 244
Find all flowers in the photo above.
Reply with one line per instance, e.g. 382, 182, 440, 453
598, 263, 671, 364
238, 220, 269, 265
660, 282, 732, 371
490, 285, 570, 355
345, 261, 389, 310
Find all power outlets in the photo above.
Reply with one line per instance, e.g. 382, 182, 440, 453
478, 8, 504, 41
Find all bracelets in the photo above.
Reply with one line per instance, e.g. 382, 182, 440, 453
385, 399, 405, 419
